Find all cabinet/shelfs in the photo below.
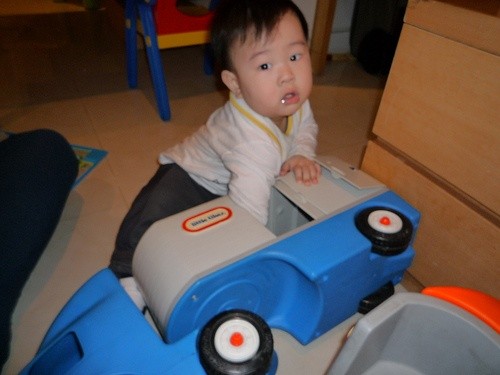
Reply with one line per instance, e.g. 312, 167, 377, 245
359, 0, 500, 295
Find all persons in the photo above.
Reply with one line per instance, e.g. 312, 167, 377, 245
0, 128, 82, 375
108, 0, 320, 279
